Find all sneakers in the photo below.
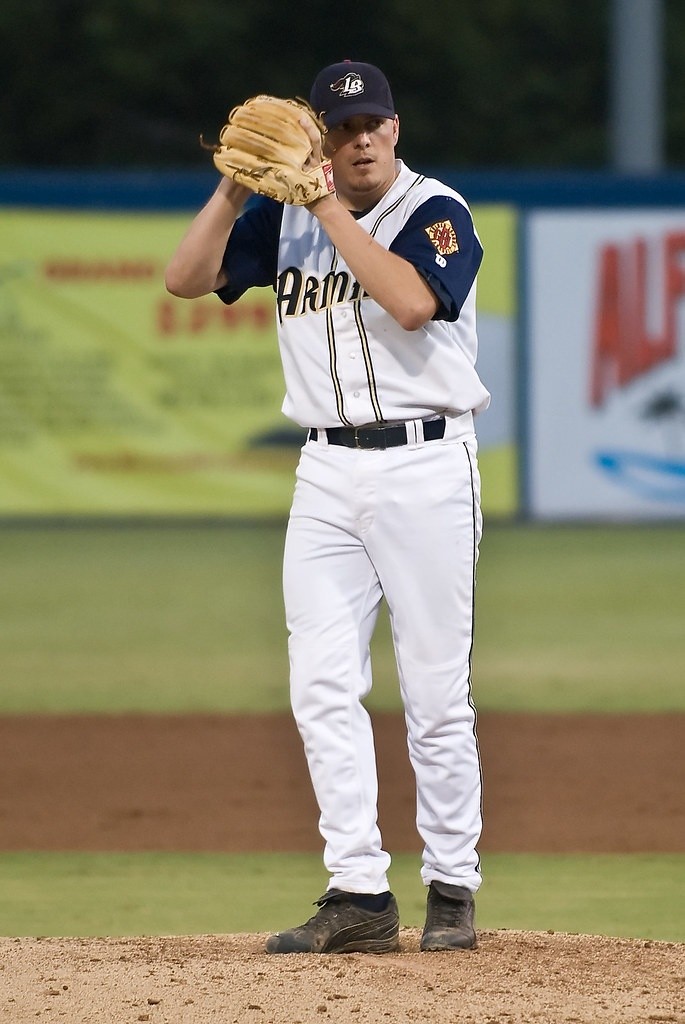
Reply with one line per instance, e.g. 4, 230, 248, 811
266, 889, 400, 954
420, 880, 476, 951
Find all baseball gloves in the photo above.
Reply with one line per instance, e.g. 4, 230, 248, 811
200, 93, 334, 206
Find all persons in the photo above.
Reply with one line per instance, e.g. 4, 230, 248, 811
163, 61, 490, 954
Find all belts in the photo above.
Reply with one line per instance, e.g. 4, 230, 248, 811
308, 418, 445, 450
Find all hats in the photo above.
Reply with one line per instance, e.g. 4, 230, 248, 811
309, 59, 394, 129
